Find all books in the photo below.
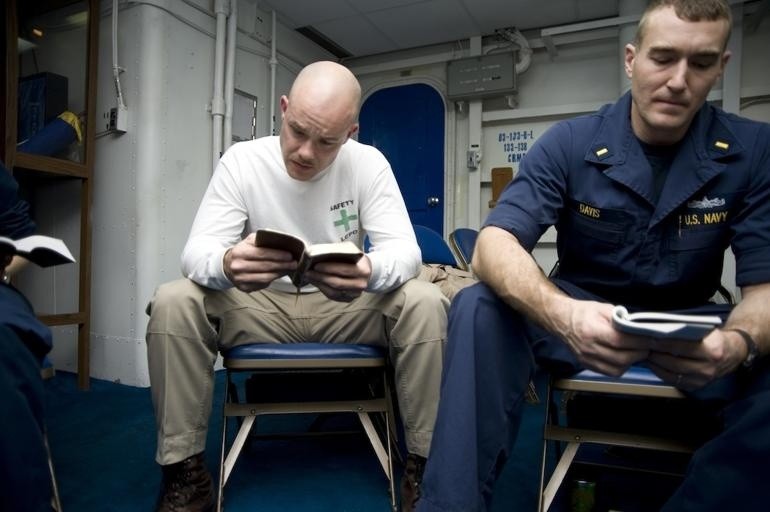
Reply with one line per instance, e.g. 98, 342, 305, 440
255, 229, 364, 288
0, 231, 76, 269
609, 304, 723, 343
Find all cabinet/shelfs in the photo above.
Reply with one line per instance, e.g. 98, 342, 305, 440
1, 0, 103, 393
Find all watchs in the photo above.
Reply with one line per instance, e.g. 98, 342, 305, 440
3, 270, 12, 284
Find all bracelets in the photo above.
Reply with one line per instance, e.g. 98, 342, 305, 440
730, 327, 758, 368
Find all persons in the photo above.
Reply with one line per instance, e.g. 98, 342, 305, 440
415, 0, 770, 512
0, 161, 53, 512
147, 60, 450, 512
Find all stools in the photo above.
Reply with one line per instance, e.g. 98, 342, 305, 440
208, 335, 421, 509
535, 360, 698, 510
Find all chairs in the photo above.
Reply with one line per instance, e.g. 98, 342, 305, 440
361, 224, 479, 272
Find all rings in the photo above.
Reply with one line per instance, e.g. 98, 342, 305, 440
677, 376, 683, 386
342, 290, 347, 300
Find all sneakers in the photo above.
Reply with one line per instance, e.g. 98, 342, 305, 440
401, 453, 426, 512
154, 451, 218, 511
